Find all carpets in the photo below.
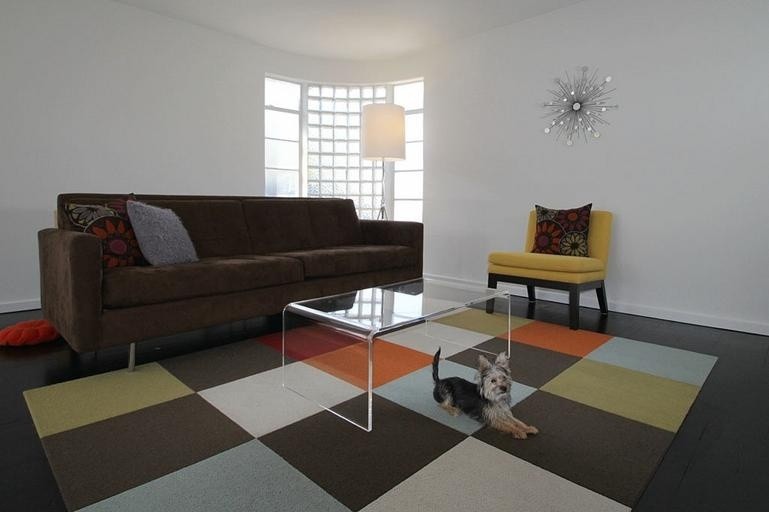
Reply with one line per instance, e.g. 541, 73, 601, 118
22, 295, 719, 511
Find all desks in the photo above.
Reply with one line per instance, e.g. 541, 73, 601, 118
283, 277, 511, 433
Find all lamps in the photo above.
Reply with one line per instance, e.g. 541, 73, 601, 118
360, 102, 406, 222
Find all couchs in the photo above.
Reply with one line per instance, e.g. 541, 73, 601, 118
41, 193, 424, 372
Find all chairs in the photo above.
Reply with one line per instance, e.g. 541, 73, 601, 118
486, 203, 613, 329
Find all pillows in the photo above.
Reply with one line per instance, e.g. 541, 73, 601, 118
530, 203, 592, 260
0, 199, 202, 349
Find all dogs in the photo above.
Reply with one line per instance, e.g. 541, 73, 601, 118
433, 347, 539, 439
